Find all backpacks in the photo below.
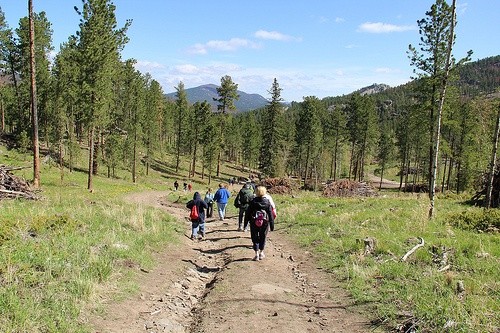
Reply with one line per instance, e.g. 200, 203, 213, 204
252, 207, 269, 227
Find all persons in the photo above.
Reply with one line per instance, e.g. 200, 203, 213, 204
205, 188, 214, 218
229, 177, 240, 186
186, 192, 208, 240
238, 178, 276, 232
188, 183, 192, 192
250, 173, 261, 183
184, 181, 187, 191
213, 183, 230, 221
244, 186, 274, 261
174, 181, 178, 190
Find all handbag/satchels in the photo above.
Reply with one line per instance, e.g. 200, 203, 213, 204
190, 204, 199, 220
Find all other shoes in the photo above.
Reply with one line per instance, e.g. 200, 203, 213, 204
191, 235, 195, 239
238, 226, 242, 230
244, 227, 247, 232
259, 254, 264, 259
253, 256, 259, 261
199, 235, 203, 239
